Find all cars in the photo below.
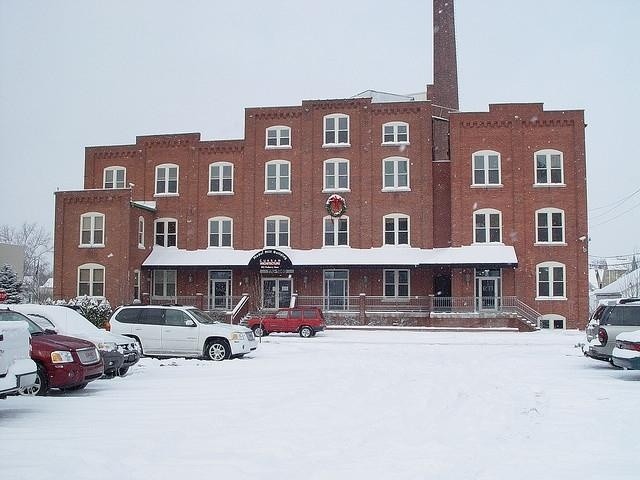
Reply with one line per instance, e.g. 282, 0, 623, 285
611, 329, 640, 371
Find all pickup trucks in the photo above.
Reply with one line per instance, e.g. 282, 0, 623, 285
0, 319, 39, 397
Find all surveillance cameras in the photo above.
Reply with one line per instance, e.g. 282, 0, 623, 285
579, 236, 586, 243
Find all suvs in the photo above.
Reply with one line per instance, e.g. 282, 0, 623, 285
106, 299, 258, 364
245, 305, 327, 337
0, 303, 105, 398
581, 294, 640, 364
9, 299, 142, 381
